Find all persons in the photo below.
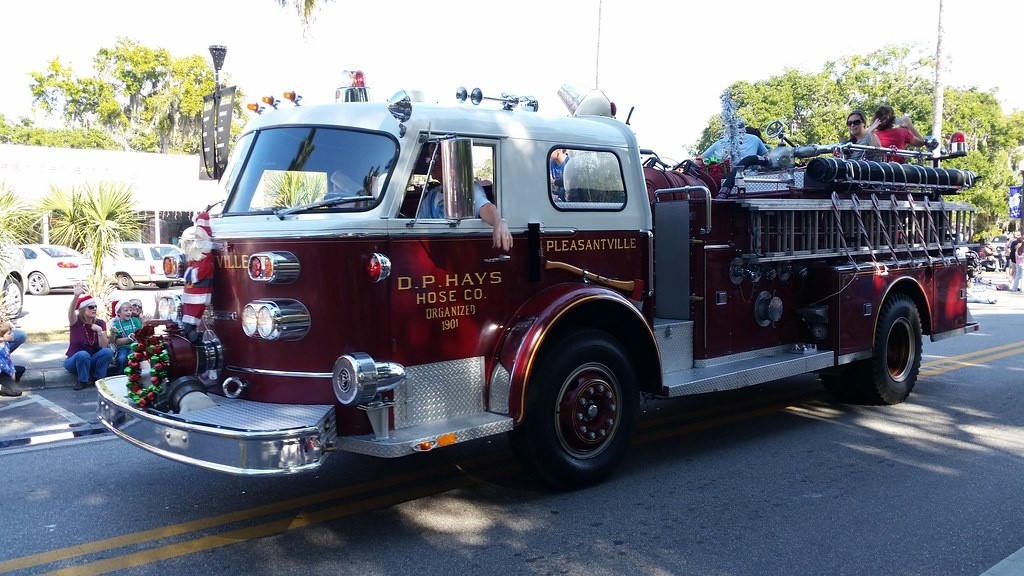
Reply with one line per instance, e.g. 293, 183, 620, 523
415, 140, 515, 252
550, 148, 571, 202
110, 299, 149, 375
0, 323, 27, 396
872, 106, 925, 163
966, 281, 997, 304
692, 119, 770, 174
845, 111, 883, 162
63, 281, 113, 390
1003, 232, 1024, 292
106, 297, 154, 342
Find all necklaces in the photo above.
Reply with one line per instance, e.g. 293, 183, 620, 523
82, 319, 96, 346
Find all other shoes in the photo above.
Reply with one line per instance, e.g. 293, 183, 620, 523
74, 381, 87, 390
92, 377, 98, 386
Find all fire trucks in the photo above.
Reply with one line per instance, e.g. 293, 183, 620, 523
95, 53, 982, 488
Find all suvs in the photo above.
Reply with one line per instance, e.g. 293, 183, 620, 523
0, 238, 27, 318
82, 242, 173, 290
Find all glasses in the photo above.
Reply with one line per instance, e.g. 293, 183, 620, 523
424, 153, 441, 164
86, 305, 97, 309
846, 120, 864, 126
132, 304, 142, 310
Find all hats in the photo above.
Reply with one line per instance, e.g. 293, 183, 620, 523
112, 301, 131, 317
75, 296, 95, 310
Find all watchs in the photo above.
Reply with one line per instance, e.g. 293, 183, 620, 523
96, 330, 104, 336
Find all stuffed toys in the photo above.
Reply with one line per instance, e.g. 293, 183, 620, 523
180, 211, 213, 346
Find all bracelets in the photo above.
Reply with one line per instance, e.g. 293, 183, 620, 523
492, 218, 509, 225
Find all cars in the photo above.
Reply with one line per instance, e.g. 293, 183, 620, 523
17, 244, 94, 295
